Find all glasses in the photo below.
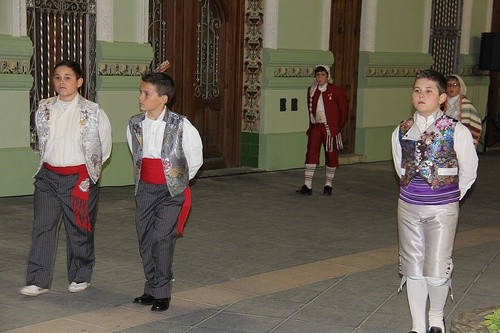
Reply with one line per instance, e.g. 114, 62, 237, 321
447, 83, 459, 87
316, 74, 325, 76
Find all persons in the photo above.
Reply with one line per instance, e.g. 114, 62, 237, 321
392, 70, 478, 333
442, 75, 483, 149
296, 64, 346, 196
126, 72, 203, 311
19, 60, 112, 295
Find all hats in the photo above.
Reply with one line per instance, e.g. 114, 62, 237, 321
448, 74, 467, 96
316, 64, 330, 80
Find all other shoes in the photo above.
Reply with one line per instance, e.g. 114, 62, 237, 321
295, 185, 312, 195
323, 185, 333, 196
20, 284, 49, 296
133, 292, 156, 305
68, 281, 90, 292
151, 294, 171, 311
427, 317, 446, 333
